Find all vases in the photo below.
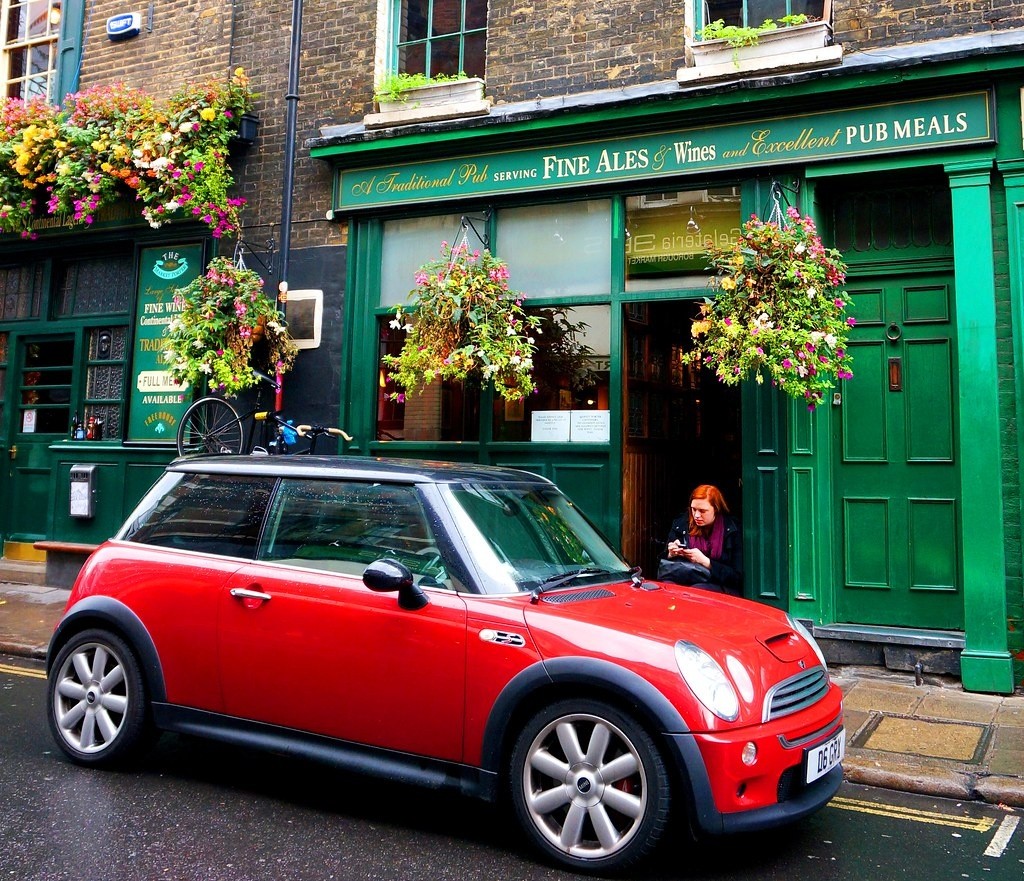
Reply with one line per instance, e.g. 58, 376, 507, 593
233, 112, 258, 141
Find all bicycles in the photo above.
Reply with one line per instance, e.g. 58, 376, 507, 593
175, 363, 354, 456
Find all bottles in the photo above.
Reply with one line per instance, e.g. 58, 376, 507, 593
70, 405, 102, 440
629, 337, 701, 440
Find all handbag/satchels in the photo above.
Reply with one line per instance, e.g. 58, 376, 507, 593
657, 558, 712, 587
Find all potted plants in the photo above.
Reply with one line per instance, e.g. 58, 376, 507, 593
691, 14, 833, 68
373, 70, 485, 112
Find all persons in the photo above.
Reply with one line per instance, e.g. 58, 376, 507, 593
656, 484, 744, 598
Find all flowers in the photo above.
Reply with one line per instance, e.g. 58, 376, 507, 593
168, 260, 297, 391
0, 68, 259, 246
686, 209, 864, 409
377, 237, 546, 407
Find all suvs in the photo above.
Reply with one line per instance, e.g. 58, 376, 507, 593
44, 451, 848, 878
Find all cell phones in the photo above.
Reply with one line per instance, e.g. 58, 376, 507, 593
678, 544, 687, 550
